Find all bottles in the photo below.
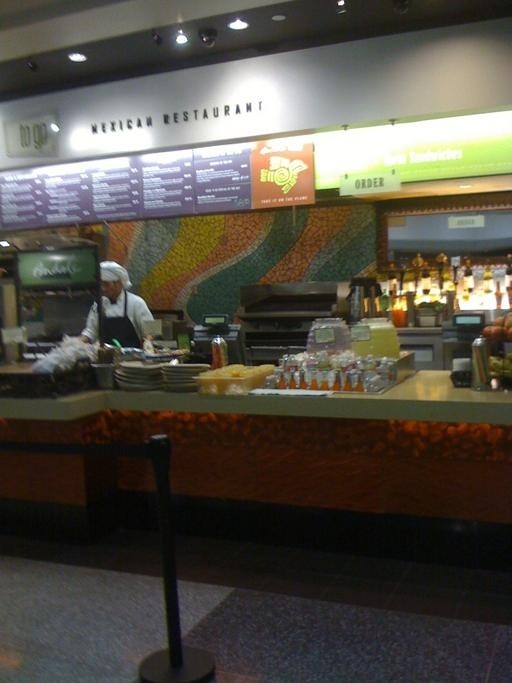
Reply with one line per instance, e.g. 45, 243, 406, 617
470, 331, 492, 392
449, 356, 471, 388
210, 335, 230, 368
346, 247, 512, 329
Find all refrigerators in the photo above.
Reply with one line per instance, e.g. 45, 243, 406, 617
12, 245, 108, 362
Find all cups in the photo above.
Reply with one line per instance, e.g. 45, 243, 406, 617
262, 344, 398, 397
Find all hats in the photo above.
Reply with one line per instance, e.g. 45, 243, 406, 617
99, 260, 120, 282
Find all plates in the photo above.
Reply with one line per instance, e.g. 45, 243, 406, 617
111, 359, 212, 393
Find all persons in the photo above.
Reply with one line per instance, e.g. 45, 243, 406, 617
76, 259, 155, 350
